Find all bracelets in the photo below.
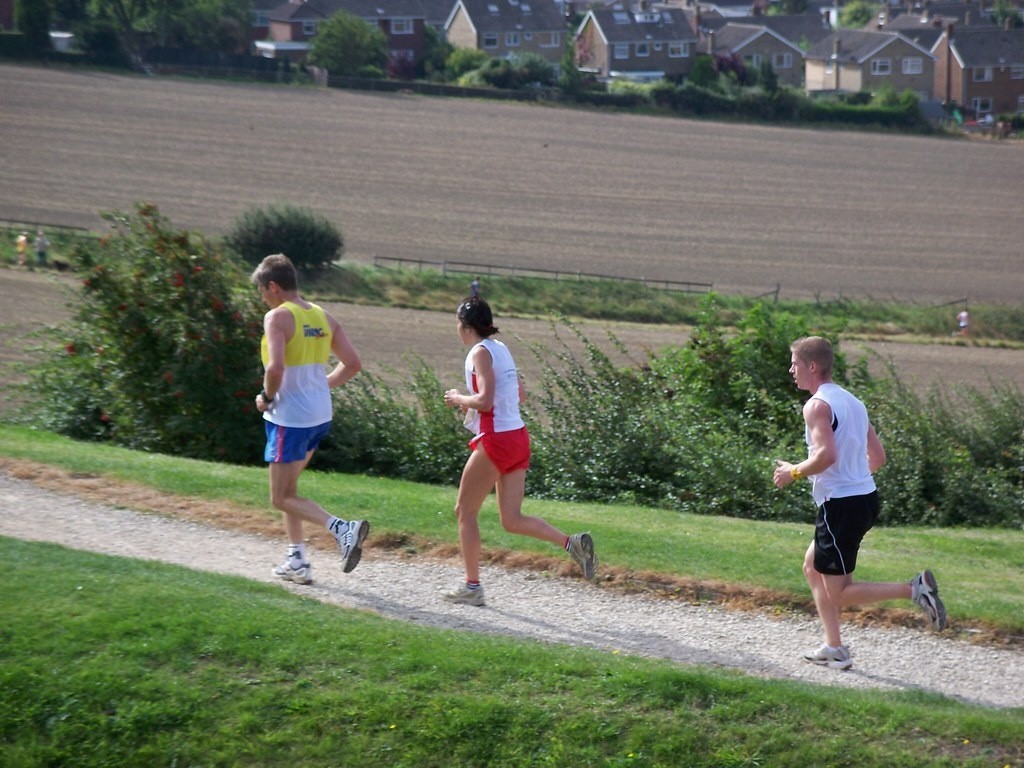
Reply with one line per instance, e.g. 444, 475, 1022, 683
261, 391, 274, 403
791, 462, 803, 481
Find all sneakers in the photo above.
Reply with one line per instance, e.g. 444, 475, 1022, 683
440, 585, 485, 606
912, 570, 945, 632
339, 520, 369, 573
802, 645, 853, 669
569, 534, 599, 581
272, 561, 312, 584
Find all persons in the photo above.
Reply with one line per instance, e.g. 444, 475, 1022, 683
251, 255, 370, 585
16, 231, 30, 267
952, 307, 971, 337
443, 295, 598, 606
773, 337, 946, 669
470, 276, 481, 297
34, 231, 50, 267
950, 103, 1011, 141
